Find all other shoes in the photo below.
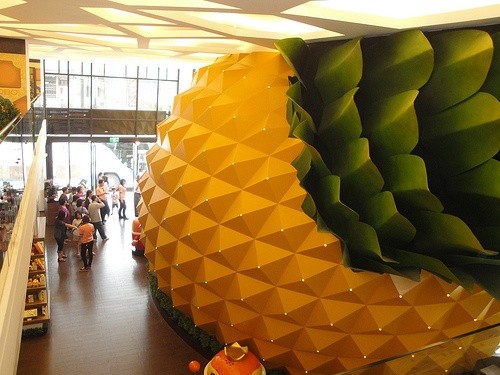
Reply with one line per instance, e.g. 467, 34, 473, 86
62, 255, 68, 258
103, 236, 109, 241
57, 257, 66, 262
119, 216, 123, 219
124, 217, 129, 220
80, 266, 89, 272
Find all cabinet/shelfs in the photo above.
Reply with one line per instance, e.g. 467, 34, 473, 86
23, 216, 51, 326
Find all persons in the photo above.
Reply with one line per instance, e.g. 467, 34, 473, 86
55, 172, 110, 262
111, 188, 119, 216
0, 189, 14, 210
134, 176, 141, 217
79, 216, 94, 272
118, 179, 128, 220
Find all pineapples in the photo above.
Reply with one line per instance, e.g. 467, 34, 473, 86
135, 27, 500, 375
